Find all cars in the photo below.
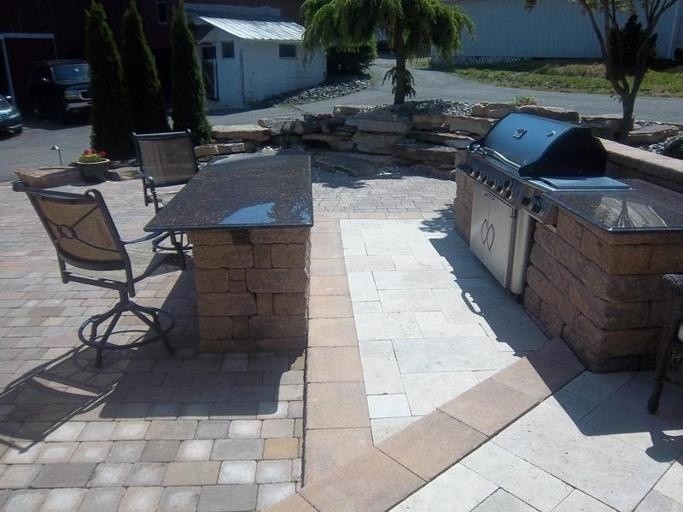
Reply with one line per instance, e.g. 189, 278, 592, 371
0, 94, 23, 138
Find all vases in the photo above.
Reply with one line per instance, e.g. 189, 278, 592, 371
69, 159, 111, 182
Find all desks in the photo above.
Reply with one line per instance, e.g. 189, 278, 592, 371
143, 149, 318, 356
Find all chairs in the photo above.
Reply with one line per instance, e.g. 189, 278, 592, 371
128, 127, 218, 270
10, 179, 177, 368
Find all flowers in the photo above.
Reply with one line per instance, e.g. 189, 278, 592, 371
81, 148, 106, 162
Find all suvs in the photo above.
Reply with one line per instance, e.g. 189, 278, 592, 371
26, 58, 97, 123
